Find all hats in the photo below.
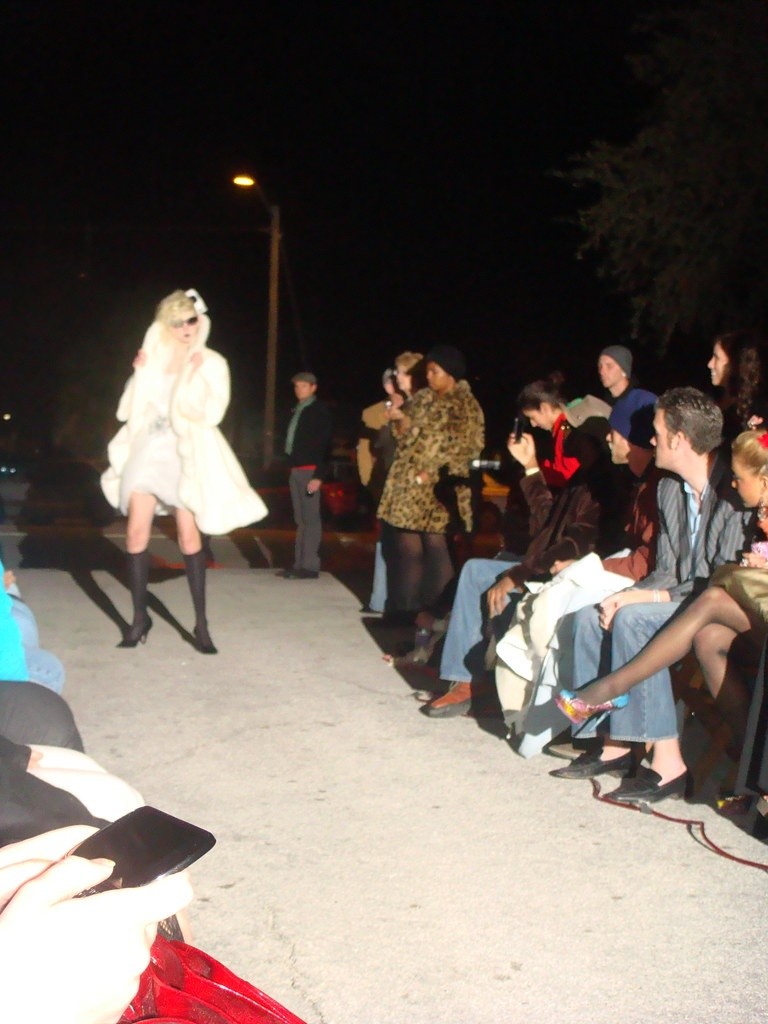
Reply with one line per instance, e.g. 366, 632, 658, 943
292, 373, 315, 383
599, 346, 633, 383
558, 395, 613, 439
610, 388, 658, 450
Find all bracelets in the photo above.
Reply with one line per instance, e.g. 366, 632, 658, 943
525, 467, 540, 475
384, 398, 392, 408
654, 590, 660, 602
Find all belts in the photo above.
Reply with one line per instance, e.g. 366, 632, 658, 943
290, 466, 317, 470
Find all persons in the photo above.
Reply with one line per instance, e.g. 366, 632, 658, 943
101, 290, 268, 652
554, 429, 768, 748
0, 562, 304, 1024
356, 326, 768, 800
283, 371, 333, 579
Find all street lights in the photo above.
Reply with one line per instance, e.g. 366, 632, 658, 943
233, 173, 284, 474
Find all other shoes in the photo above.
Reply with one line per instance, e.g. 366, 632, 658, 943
276, 568, 319, 579
547, 740, 591, 760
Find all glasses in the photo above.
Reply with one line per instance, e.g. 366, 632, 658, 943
560, 422, 571, 431
170, 316, 199, 329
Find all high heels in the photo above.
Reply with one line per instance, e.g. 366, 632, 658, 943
716, 794, 752, 815
555, 689, 630, 735
195, 625, 217, 654
117, 617, 153, 647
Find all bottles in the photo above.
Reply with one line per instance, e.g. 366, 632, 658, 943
413, 625, 430, 665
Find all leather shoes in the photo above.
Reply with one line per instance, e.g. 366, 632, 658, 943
601, 767, 694, 803
426, 678, 502, 718
549, 748, 636, 780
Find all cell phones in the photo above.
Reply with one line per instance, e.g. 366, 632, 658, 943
514, 418, 524, 444
69, 806, 217, 899
